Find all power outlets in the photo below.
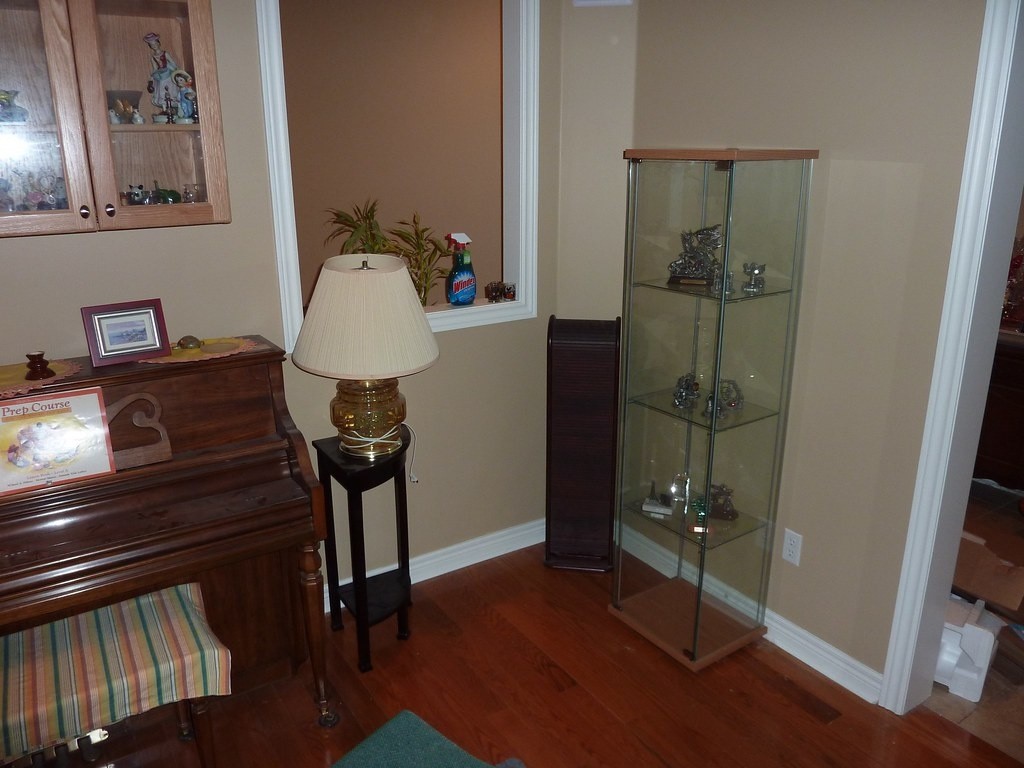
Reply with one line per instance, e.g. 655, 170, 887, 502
782, 527, 803, 567
756, 515, 779, 554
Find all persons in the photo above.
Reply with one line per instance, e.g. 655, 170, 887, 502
144, 34, 196, 118
36, 167, 68, 210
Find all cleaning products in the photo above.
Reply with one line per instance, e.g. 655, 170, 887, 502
445, 232, 477, 306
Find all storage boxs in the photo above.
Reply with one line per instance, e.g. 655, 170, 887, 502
933, 593, 1005, 704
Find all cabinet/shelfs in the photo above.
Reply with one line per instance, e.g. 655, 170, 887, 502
0, 0, 231, 238
606, 146, 821, 674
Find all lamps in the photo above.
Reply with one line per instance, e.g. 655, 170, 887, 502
290, 253, 441, 461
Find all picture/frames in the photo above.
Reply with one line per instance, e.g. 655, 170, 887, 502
80, 298, 172, 368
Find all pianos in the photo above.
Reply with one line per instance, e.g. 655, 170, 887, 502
1, 334, 342, 768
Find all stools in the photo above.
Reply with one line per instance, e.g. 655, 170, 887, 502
308, 423, 413, 670
0, 584, 233, 768
